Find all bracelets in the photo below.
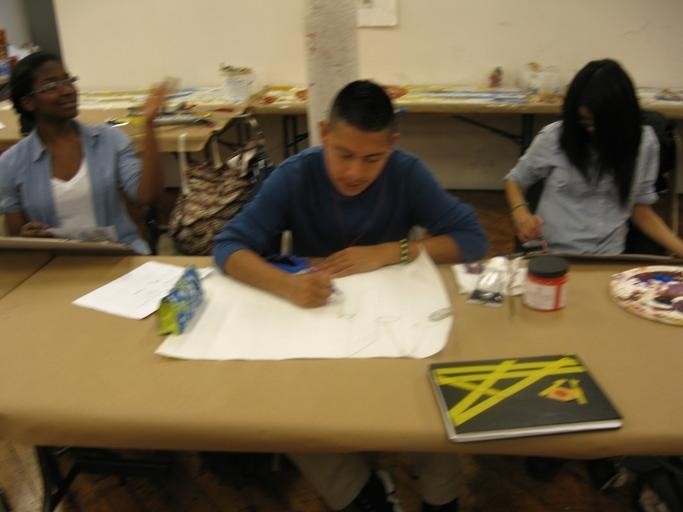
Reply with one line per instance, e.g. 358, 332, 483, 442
396, 237, 409, 266
507, 202, 531, 213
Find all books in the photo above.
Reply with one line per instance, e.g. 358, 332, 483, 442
425, 351, 624, 444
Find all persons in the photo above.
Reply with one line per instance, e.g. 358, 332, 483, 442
209, 77, 490, 510
500, 55, 681, 260
0, 49, 178, 262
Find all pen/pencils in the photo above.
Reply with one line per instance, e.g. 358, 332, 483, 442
289, 254, 343, 296
532, 215, 549, 253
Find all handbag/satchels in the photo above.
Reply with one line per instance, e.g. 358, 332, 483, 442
167, 112, 274, 256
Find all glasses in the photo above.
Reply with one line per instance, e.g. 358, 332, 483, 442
25, 76, 80, 98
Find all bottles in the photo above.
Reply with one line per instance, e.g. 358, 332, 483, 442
522, 255, 572, 311
